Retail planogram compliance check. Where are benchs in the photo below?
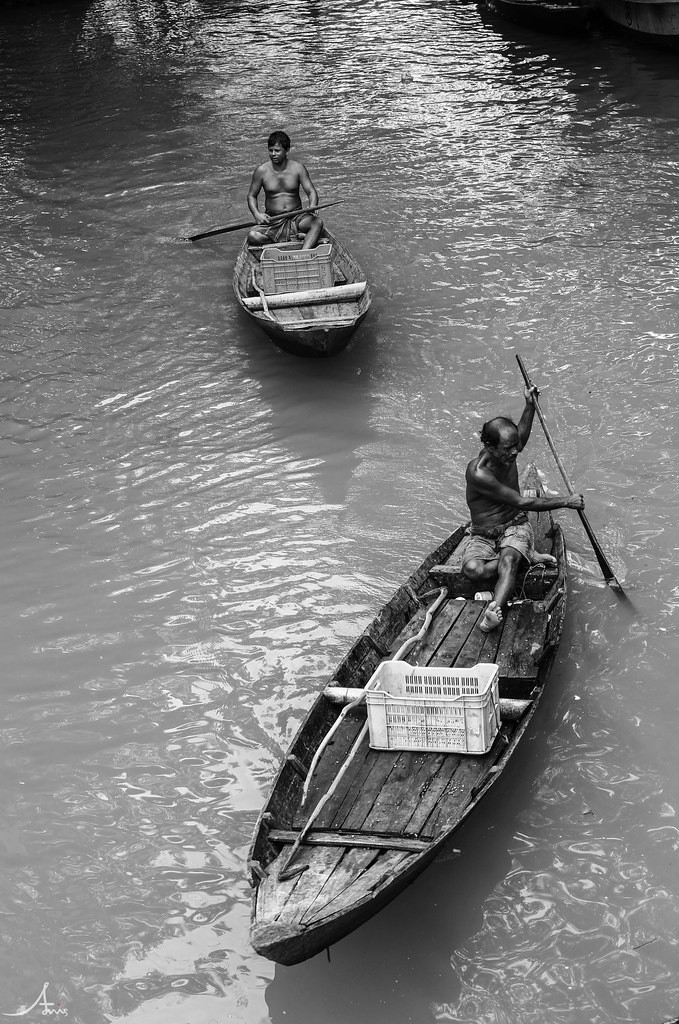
[243,281,366,314]
[248,237,327,264]
[430,558,558,579]
[323,683,532,718]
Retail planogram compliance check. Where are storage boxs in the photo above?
[261,243,332,296]
[365,661,500,754]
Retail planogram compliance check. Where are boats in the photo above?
[243,459,569,968]
[232,211,373,358]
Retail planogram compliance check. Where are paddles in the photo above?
[187,198,347,241]
[514,353,635,606]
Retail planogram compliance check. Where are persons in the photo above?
[246,131,325,260]
[459,379,586,633]
